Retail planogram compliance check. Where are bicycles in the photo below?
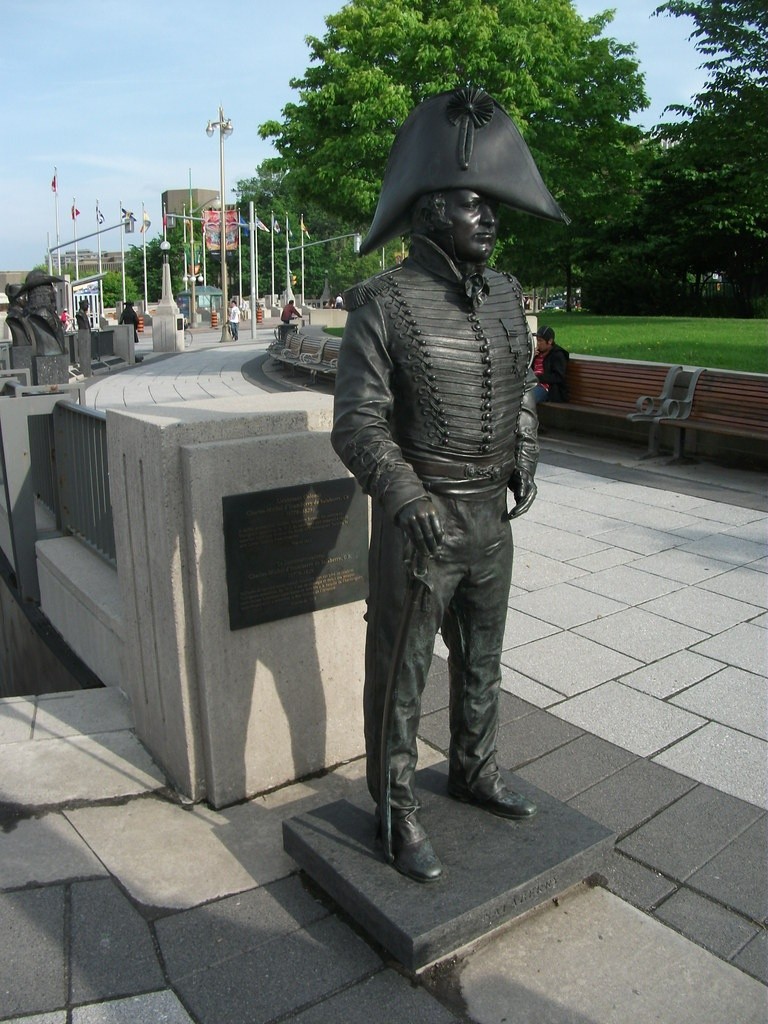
[184,329,193,348]
[273,316,303,342]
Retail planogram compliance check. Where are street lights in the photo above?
[205,102,234,343]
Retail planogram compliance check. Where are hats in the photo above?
[531,326,556,340]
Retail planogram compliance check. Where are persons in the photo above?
[227,300,240,340]
[527,326,570,405]
[61,308,70,332]
[119,301,143,363]
[332,86,573,886]
[76,299,91,332]
[329,292,344,308]
[281,300,303,324]
[4,270,69,356]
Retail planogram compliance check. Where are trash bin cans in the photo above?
[151,314,185,352]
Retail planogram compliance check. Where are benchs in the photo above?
[537,352,683,461]
[652,368,768,468]
[265,324,343,388]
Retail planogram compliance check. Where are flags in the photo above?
[122,207,137,221]
[186,219,191,231]
[51,171,56,193]
[95,204,105,224]
[239,216,249,237]
[288,223,293,237]
[257,217,270,232]
[141,209,151,232]
[72,205,80,219]
[300,219,310,239]
[273,218,280,233]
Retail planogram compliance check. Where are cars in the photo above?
[542,299,574,311]
